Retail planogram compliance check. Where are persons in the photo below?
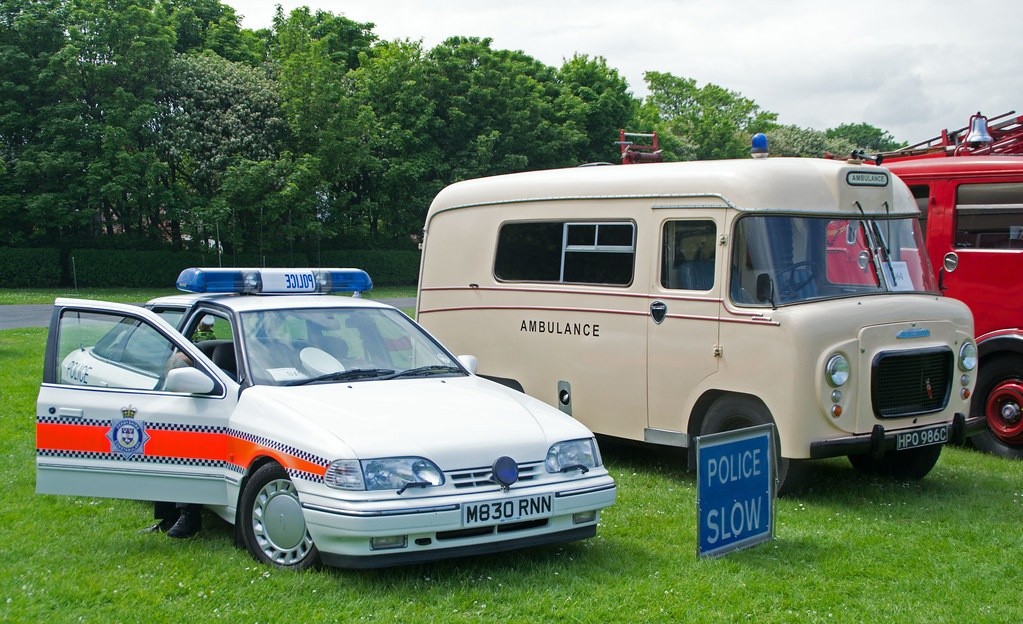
[142,352,238,537]
[191,315,218,342]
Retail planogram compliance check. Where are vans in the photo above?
[410,130,981,478]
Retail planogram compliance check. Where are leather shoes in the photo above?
[139,518,176,536]
[165,514,201,539]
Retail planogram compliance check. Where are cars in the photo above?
[33,269,621,570]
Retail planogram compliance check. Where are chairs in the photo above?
[678,260,740,302]
[784,269,818,301]
[211,342,236,374]
[320,336,349,361]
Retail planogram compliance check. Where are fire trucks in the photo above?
[820,109,1023,447]
